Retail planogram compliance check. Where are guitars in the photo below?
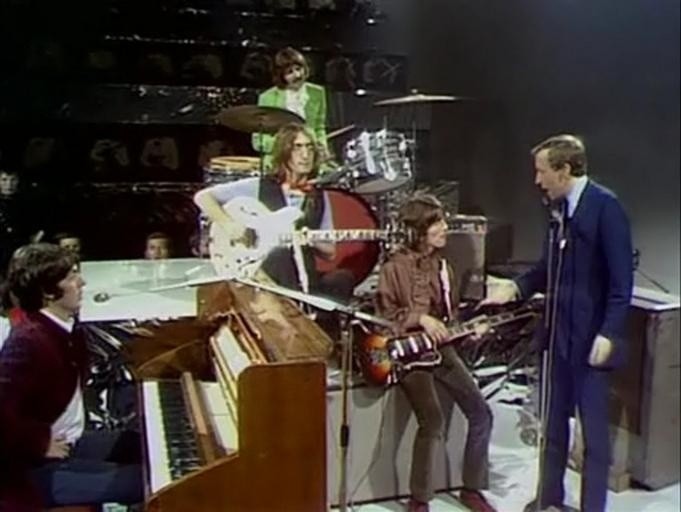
[359,298,547,385]
[211,196,393,280]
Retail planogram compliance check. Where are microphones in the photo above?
[91,285,134,305]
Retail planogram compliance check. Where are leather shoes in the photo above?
[408,499,429,512]
[461,489,497,512]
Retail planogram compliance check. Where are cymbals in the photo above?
[372,92,459,107]
[218,104,307,133]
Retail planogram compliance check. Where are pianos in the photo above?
[132,279,335,507]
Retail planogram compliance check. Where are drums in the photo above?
[343,130,413,192]
[205,156,264,183]
[313,189,380,288]
[444,212,488,301]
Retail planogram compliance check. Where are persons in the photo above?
[376,193,498,511]
[193,123,338,323]
[0,244,141,512]
[1,1,406,258]
[475,135,636,512]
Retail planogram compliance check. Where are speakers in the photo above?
[575,286,681,490]
